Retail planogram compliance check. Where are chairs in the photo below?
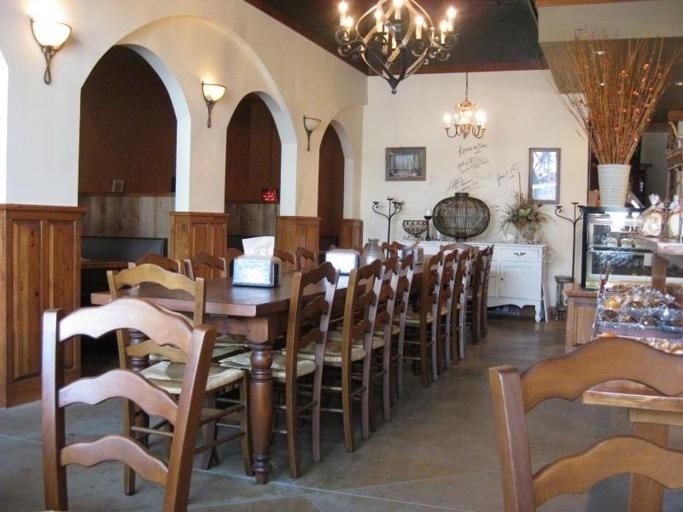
[489,337,683,512]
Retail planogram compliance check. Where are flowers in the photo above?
[500,193,554,227]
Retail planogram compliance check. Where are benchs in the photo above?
[81,235,168,289]
[319,236,339,263]
[227,235,261,276]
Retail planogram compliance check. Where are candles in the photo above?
[425,208,432,215]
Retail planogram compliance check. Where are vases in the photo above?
[526,227,544,245]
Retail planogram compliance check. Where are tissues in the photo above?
[232,233,281,289]
[325,248,359,274]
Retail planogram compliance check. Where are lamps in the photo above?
[334,0,488,139]
[30,19,72,84]
[201,83,225,128]
[261,118,280,203]
[302,116,322,151]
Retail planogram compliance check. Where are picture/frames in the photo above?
[385,146,426,180]
[112,179,124,193]
[528,147,560,204]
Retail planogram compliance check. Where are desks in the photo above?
[582,379,683,511]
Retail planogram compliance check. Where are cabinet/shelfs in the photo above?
[397,240,549,322]
[566,111,683,354]
